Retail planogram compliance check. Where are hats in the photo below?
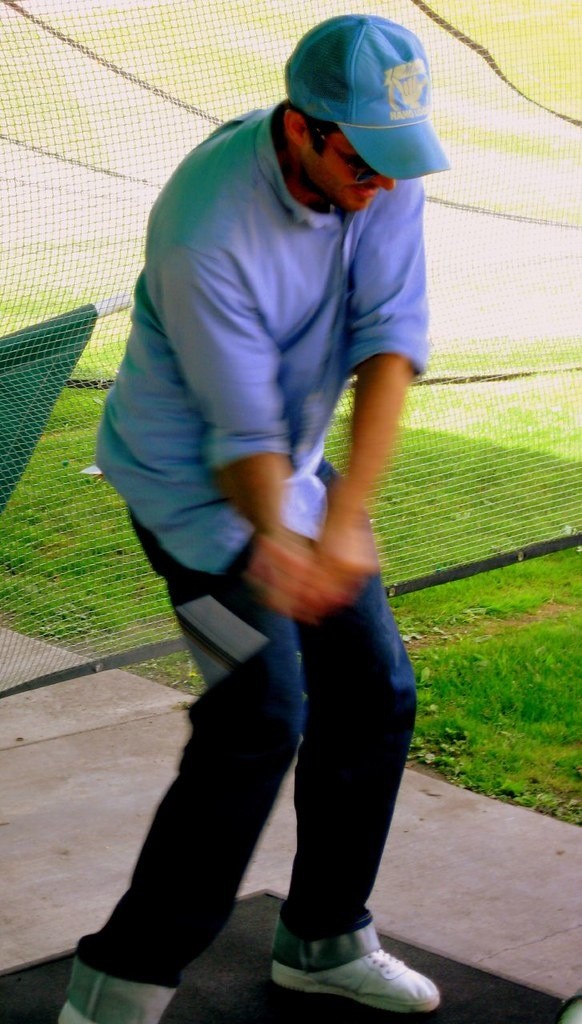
[284,14,452,180]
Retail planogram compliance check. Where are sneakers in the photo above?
[271,947,440,1015]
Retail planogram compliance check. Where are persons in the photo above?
[55,12,455,1024]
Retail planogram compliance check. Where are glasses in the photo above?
[312,124,381,184]
